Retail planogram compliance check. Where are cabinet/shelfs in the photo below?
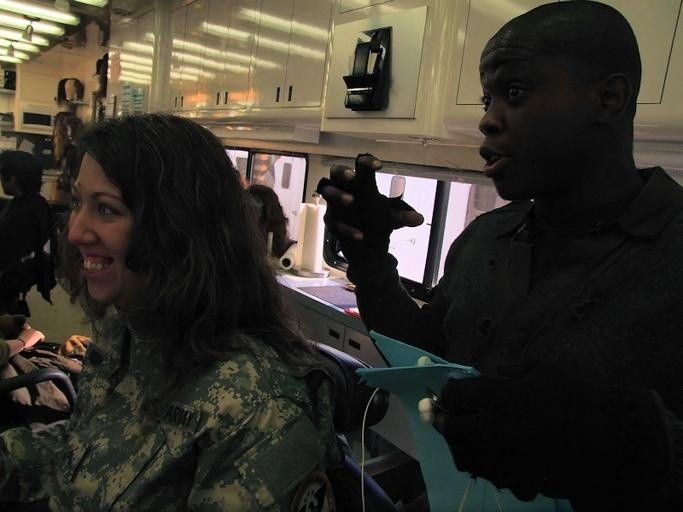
[15,20,102,117]
[162,0,209,126]
[434,0,683,145]
[278,286,389,371]
[209,0,255,120]
[255,0,336,114]
[106,3,162,140]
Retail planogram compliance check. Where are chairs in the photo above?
[297,337,402,508]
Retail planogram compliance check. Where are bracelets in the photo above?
[17,337,25,350]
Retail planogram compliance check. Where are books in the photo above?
[354,329,572,512]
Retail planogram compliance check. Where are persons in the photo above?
[53,110,87,182]
[0,320,71,415]
[0,112,336,512]
[316,0,682,505]
[246,182,290,249]
[0,148,53,265]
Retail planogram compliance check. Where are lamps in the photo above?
[20,14,42,42]
